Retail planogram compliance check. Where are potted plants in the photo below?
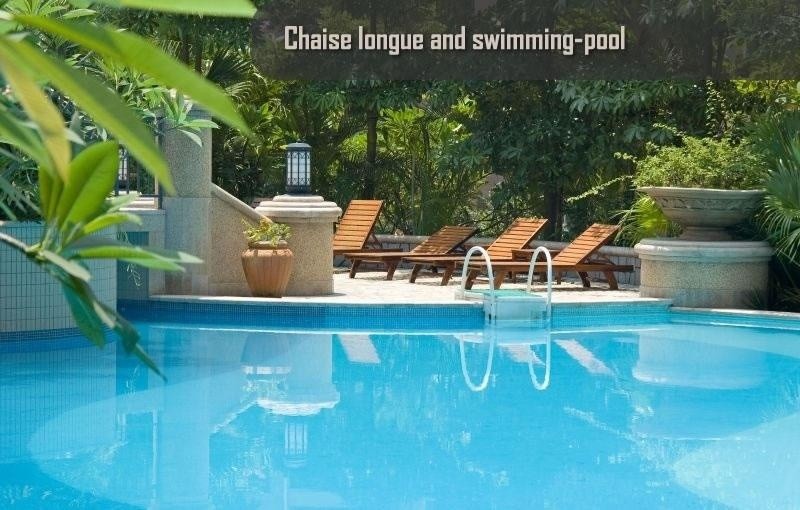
[240,216,293,297]
[564,81,767,243]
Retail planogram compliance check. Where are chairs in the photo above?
[337,334,381,365]
[503,343,543,365]
[333,198,634,291]
[556,338,607,371]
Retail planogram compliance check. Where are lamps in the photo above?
[283,417,309,469]
[116,143,129,186]
[285,142,312,194]
